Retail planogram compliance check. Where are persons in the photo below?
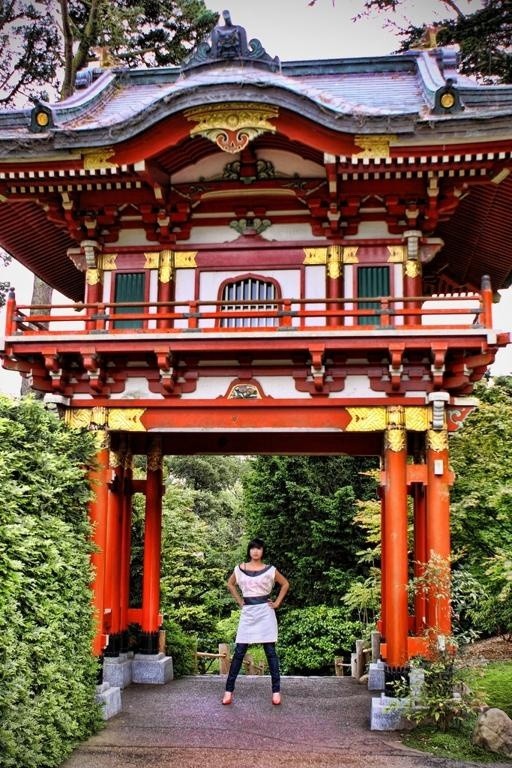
[219,537,291,706]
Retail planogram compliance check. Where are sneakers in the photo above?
[222,695,232,704]
[273,694,281,705]
